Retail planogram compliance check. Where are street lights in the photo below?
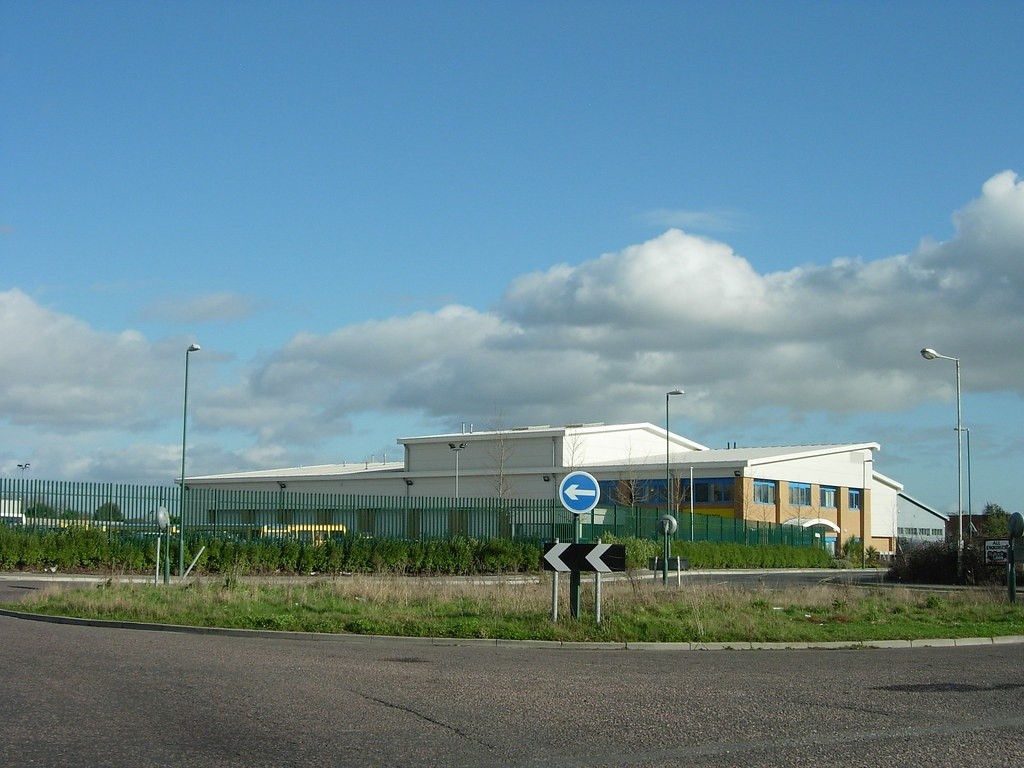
[919,346,965,553]
[448,441,467,498]
[666,389,685,557]
[954,427,975,547]
[176,342,202,580]
